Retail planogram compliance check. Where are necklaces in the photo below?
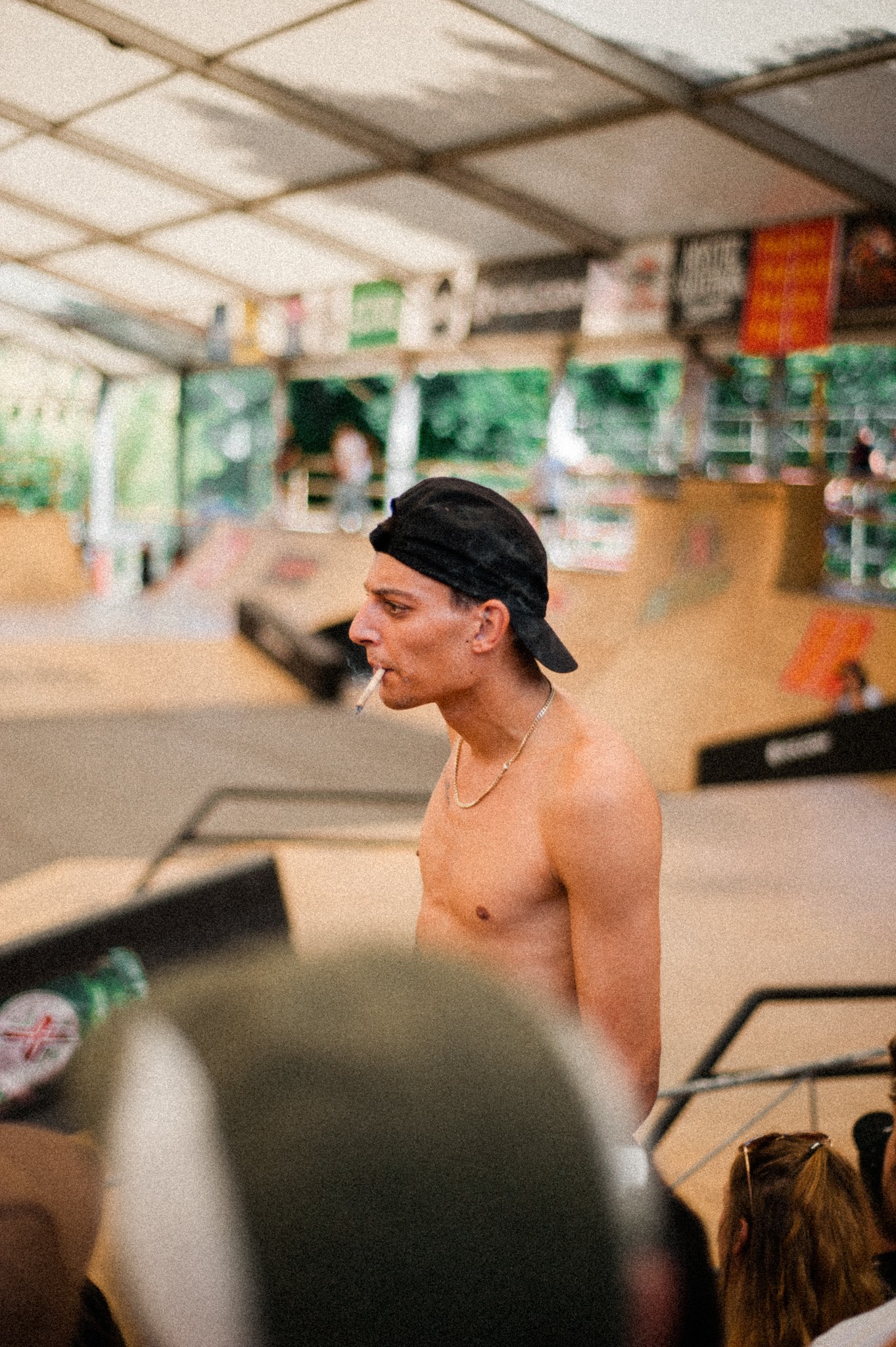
[453,675,555,809]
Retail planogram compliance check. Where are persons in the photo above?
[76,931,670,1347]
[349,480,664,1139]
[820,662,879,716]
[714,1133,885,1346]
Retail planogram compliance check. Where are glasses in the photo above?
[738,1130,830,1216]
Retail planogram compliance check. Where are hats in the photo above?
[62,937,636,1345]
[367,476,580,676]
